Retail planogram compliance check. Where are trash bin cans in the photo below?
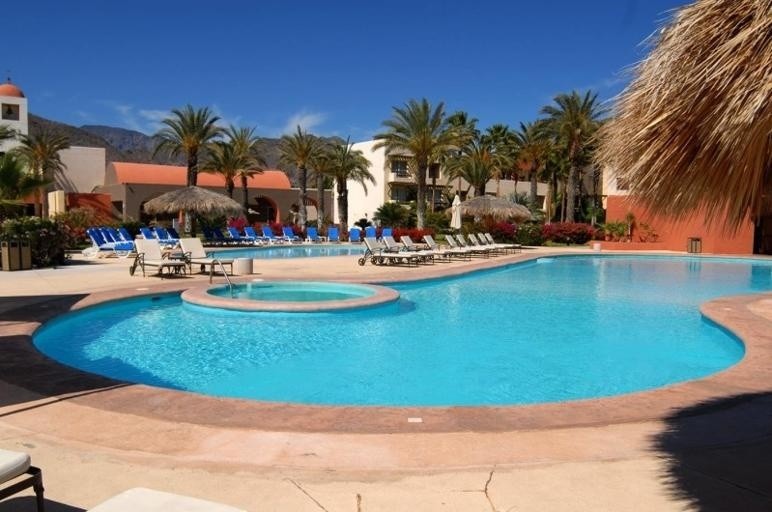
[687,236,702,253]
[1,239,33,271]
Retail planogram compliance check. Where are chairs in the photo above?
[228,224,392,249]
[86,224,236,279]
[356,229,522,267]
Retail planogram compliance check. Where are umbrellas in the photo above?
[450,195,461,230]
